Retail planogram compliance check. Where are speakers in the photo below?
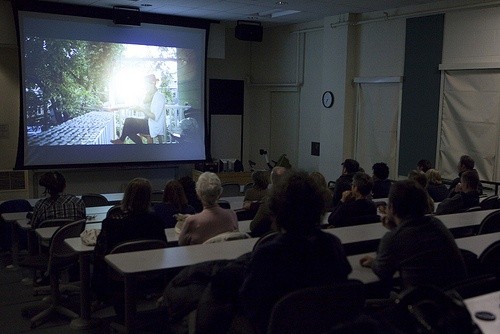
[114,9,141,26]
[235,24,264,41]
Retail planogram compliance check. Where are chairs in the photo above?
[268,284,355,334]
[20,218,86,331]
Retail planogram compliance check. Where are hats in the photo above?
[341,159,359,171]
[141,74,160,83]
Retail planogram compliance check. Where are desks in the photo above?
[0,169,500,334]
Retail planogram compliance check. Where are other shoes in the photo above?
[110,139,124,144]
[157,297,167,307]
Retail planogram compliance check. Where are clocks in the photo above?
[322,91,334,108]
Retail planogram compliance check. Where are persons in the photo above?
[151,178,196,228]
[18,171,88,281]
[359,180,472,301]
[436,170,480,215]
[233,167,353,334]
[110,74,166,144]
[177,176,203,214]
[242,154,483,235]
[90,177,168,319]
[178,171,239,247]
[328,172,377,228]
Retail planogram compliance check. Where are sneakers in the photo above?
[23,274,43,286]
